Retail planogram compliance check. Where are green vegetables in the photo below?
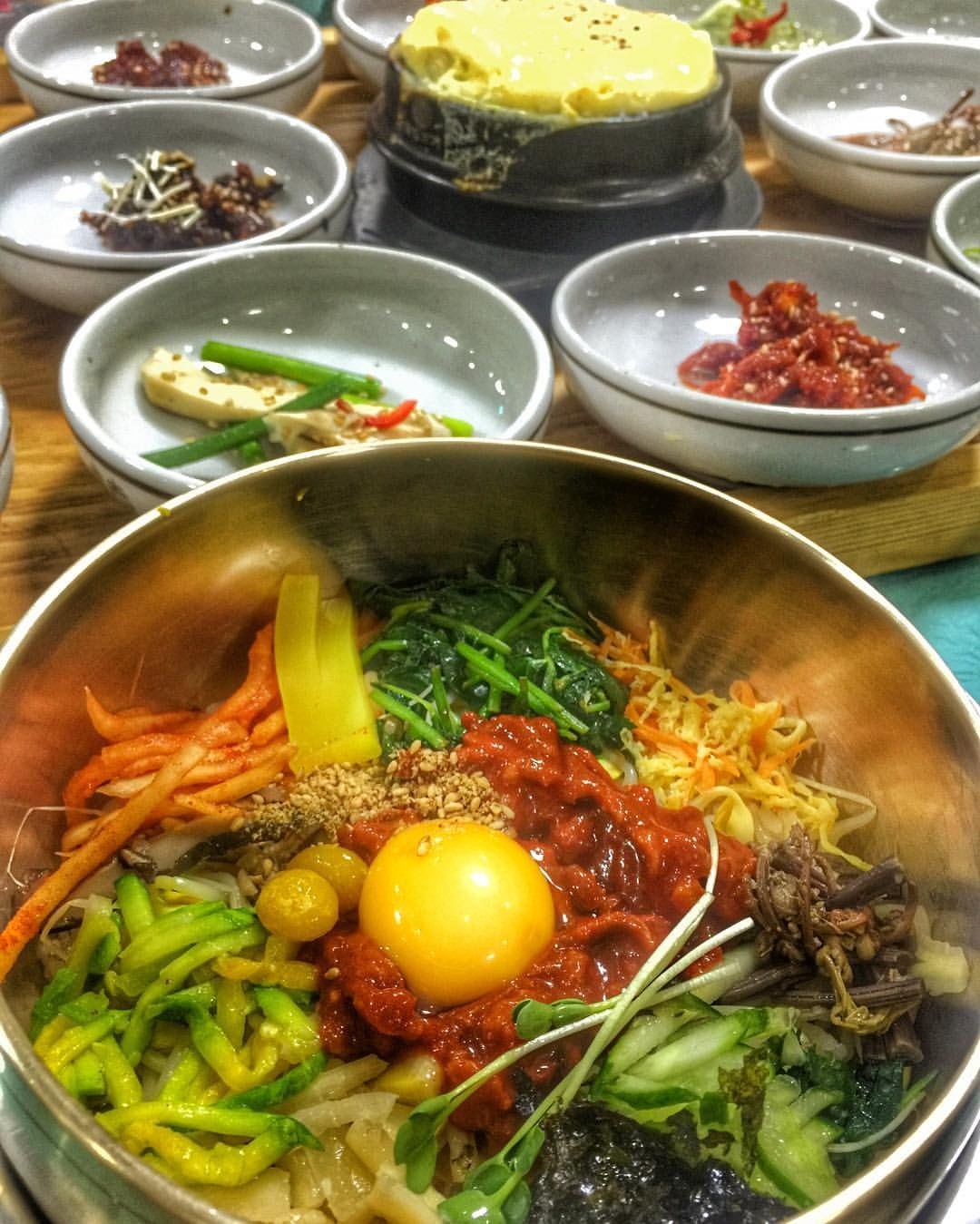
[134,337,383,469]
[341,535,638,754]
[393,811,939,1223]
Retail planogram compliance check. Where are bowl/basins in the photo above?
[1,439,980,1222]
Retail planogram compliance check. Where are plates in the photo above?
[624,0,871,111]
[871,0,980,282]
[0,100,359,314]
[760,36,980,219]
[337,0,422,95]
[554,227,980,485]
[7,2,324,114]
[59,239,554,517]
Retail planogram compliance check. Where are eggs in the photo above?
[359,816,557,1013]
